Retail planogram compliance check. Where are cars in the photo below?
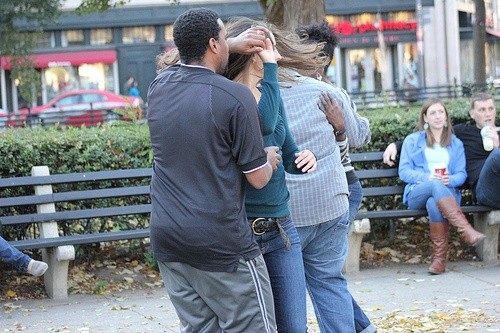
[7,91,143,127]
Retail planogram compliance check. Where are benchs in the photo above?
[0,152,500,297]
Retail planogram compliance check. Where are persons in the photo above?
[123,75,143,103]
[147,9,282,333]
[256,67,371,333]
[398,97,486,275]
[296,22,379,333]
[155,16,331,333]
[383,92,500,210]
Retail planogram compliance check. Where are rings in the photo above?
[257,30,260,35]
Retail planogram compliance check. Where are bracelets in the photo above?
[333,128,346,137]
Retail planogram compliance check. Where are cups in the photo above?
[480,126,494,151]
[434,168,446,176]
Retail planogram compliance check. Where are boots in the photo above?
[437,196,486,246]
[429,222,448,274]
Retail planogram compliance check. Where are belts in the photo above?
[346,170,355,178]
[249,217,291,250]
